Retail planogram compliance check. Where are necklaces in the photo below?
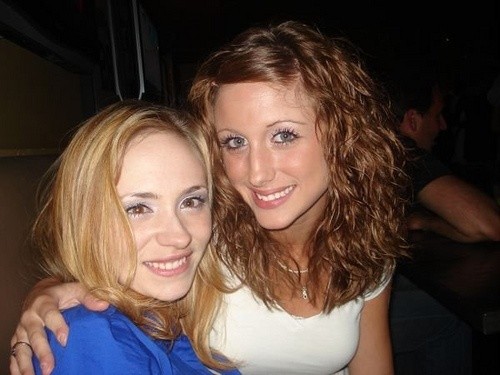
[273,255,326,302]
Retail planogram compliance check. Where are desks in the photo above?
[397,230,499,309]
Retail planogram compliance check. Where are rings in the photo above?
[10,341,35,361]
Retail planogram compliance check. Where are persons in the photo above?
[10,21,417,375]
[381,68,500,375]
[31,102,242,375]
